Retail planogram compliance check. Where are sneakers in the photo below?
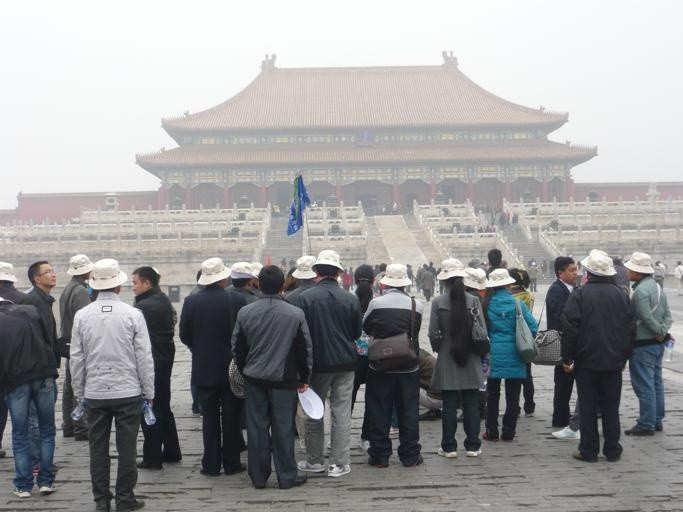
[11,486,32,498]
[327,462,353,478]
[39,481,58,494]
[297,458,325,473]
[572,448,597,463]
[465,447,482,457]
[625,423,654,437]
[437,447,458,458]
[94,500,111,511]
[651,422,663,433]
[404,454,424,466]
[367,457,389,468]
[551,426,581,440]
[419,409,441,422]
[32,465,59,477]
[482,432,499,442]
[359,437,371,452]
[115,498,147,512]
[198,410,222,418]
[0,447,6,458]
[525,409,535,417]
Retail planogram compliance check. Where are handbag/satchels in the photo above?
[53,333,70,359]
[512,307,534,365]
[367,333,419,374]
[470,302,490,358]
[530,329,563,365]
[228,358,245,401]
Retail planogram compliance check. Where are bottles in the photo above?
[479,352,488,392]
[71,402,85,421]
[141,399,157,426]
[663,337,673,362]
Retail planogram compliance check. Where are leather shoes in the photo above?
[136,460,161,470]
[198,465,221,476]
[225,461,247,475]
[161,454,183,461]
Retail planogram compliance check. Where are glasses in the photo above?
[37,267,54,279]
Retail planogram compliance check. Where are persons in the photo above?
[476,198,509,234]
[0,254,181,510]
[179,250,682,490]
[378,199,399,214]
[273,197,293,212]
[312,201,318,207]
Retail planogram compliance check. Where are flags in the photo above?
[286,176,310,237]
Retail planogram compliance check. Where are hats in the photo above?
[229,262,258,279]
[622,252,656,275]
[486,266,518,288]
[296,387,325,423]
[579,248,618,278]
[88,257,128,291]
[250,262,265,277]
[65,253,94,277]
[0,261,19,283]
[379,262,412,288]
[292,254,318,280]
[197,256,232,286]
[436,258,467,281]
[312,249,345,274]
[462,267,488,291]
